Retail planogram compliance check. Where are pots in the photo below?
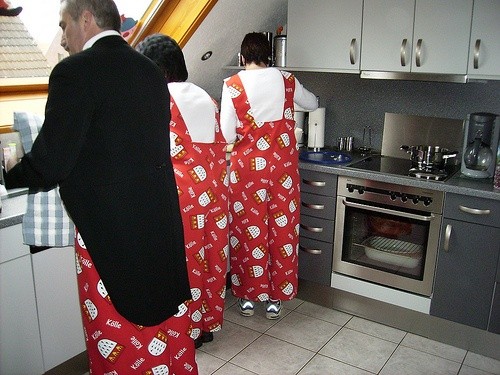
[399,143,460,178]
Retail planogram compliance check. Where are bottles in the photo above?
[338,136,354,152]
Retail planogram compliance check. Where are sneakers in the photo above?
[237,297,255,316]
[265,298,283,319]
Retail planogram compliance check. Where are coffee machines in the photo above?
[460,112,500,179]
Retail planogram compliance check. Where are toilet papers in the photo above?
[307,107,326,148]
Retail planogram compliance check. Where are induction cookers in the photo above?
[342,111,466,184]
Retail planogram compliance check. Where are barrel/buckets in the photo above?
[274,35,286,68]
[259,32,272,66]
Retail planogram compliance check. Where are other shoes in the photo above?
[200,331,213,342]
[194,338,202,349]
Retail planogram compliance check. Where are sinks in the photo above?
[342,155,456,188]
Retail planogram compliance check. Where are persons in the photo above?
[219,33,318,319]
[134,33,229,349]
[0,0,197,374]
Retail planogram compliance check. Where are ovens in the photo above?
[329,175,447,316]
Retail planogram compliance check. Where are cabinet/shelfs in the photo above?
[0,221,87,375]
[357,0,474,74]
[430,193,500,331]
[294,173,336,285]
[467,0,500,78]
[282,0,362,71]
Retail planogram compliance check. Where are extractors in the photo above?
[359,70,472,85]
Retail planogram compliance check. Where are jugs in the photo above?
[464,137,493,170]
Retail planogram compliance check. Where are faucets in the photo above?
[359,125,368,156]
